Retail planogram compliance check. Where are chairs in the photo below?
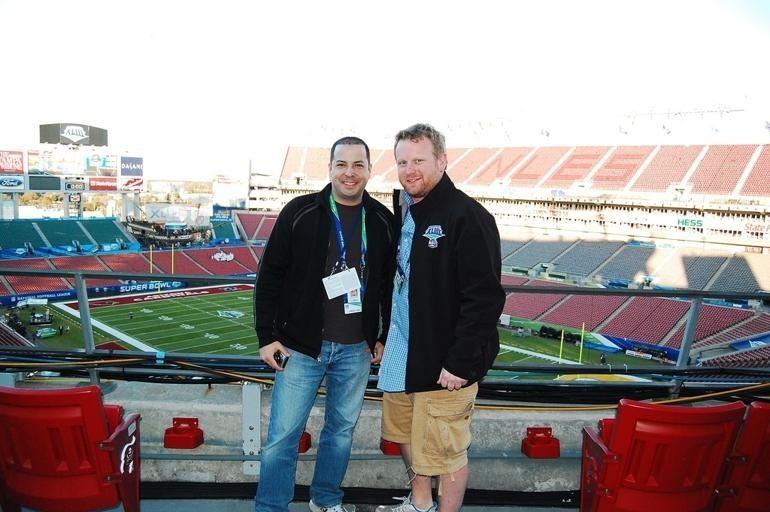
[579,398,770,512]
[0,386,142,512]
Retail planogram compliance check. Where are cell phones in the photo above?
[273,350,289,369]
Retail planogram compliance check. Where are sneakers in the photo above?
[375,493,437,512]
[309,499,343,512]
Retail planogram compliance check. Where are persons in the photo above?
[599,353,606,365]
[700,351,703,358]
[8,313,27,337]
[253,135,394,512]
[687,356,691,365]
[373,123,507,512]
[33,334,35,341]
[31,306,36,316]
[128,312,134,323]
[58,324,64,335]
[696,355,699,363]
[64,324,70,335]
